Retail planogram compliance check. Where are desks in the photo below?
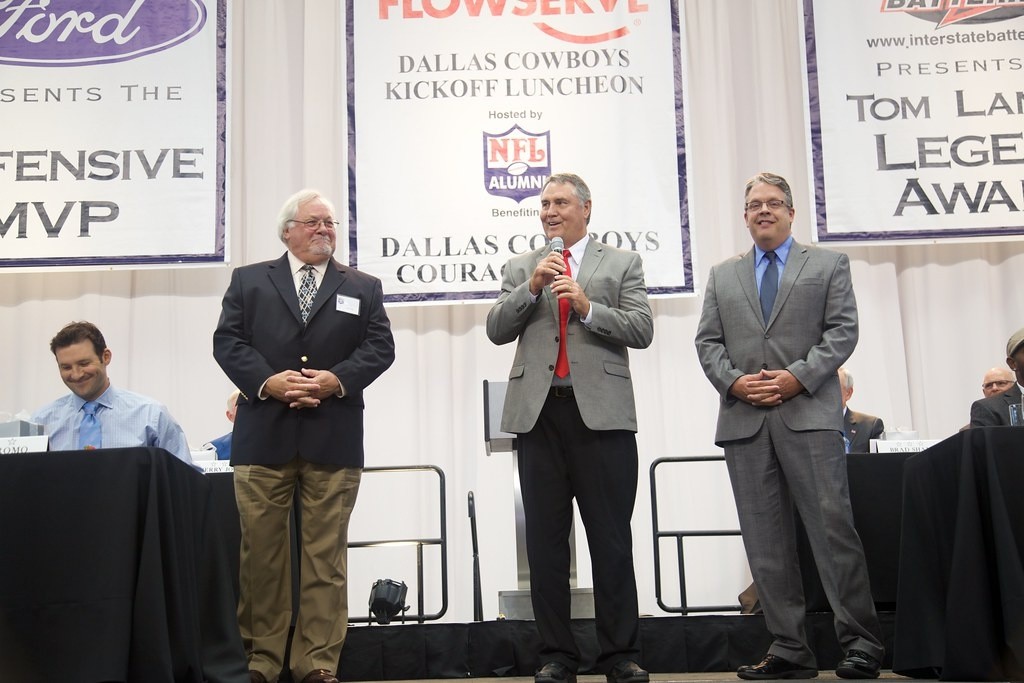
[795,425,1024,683]
[0,446,300,683]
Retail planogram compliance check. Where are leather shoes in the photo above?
[606,660,650,683]
[534,661,577,683]
[303,668,339,683]
[835,649,881,678]
[737,653,819,680]
[249,670,267,683]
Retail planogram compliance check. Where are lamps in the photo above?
[368,579,407,626]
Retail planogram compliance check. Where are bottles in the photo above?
[843,432,849,453]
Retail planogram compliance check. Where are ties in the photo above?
[77,401,102,450]
[554,250,572,378]
[760,252,779,327]
[298,265,318,328]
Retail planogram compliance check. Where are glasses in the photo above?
[746,200,791,211]
[982,380,1015,389]
[287,220,339,230]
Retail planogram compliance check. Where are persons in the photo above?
[212,189,396,683]
[969,327,1024,427]
[834,368,884,452]
[29,322,193,464]
[486,173,653,682]
[203,391,241,460]
[982,367,1015,397]
[695,173,886,680]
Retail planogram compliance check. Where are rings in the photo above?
[568,286,571,292]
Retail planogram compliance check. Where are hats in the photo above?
[1007,328,1024,357]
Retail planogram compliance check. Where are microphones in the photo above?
[551,236,564,281]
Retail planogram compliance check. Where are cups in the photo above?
[1008,403,1024,426]
